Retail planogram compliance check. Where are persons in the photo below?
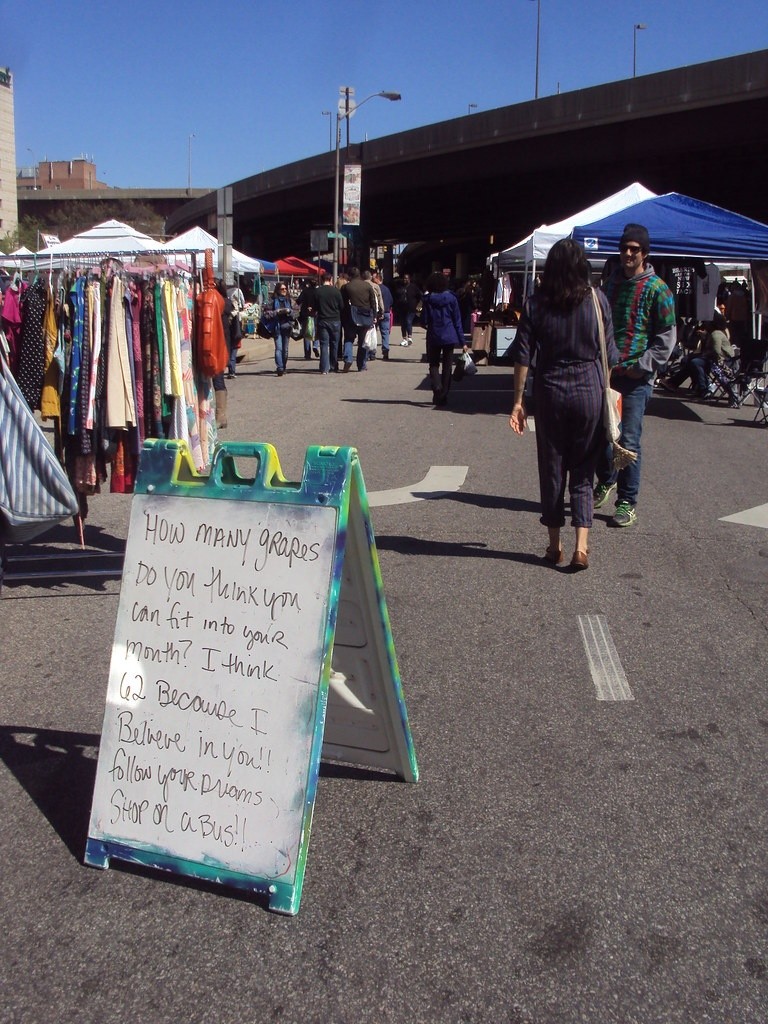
[509,239,617,570]
[592,223,677,527]
[659,311,731,399]
[213,279,245,429]
[251,267,393,376]
[395,268,468,405]
[344,204,359,223]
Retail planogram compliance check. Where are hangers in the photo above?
[0,249,215,284]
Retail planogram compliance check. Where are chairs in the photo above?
[654,345,768,426]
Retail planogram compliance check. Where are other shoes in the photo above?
[400,339,409,347]
[277,371,283,376]
[432,388,440,405]
[545,547,565,565]
[312,347,320,357]
[228,374,235,379]
[437,401,447,407]
[659,382,677,394]
[408,338,413,346]
[570,551,589,569]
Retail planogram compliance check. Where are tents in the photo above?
[0,219,325,318]
[568,192,768,339]
[494,182,755,340]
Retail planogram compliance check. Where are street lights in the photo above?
[189,133,196,189]
[632,23,648,79]
[26,148,37,191]
[468,103,478,115]
[331,89,402,286]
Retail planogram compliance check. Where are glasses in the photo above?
[617,244,643,254]
[280,288,288,290]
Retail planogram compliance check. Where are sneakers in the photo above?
[610,499,637,527]
[593,481,617,508]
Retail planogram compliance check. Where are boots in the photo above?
[215,389,228,431]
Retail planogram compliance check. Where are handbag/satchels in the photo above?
[602,387,638,469]
[361,323,377,355]
[453,358,466,382]
[305,317,315,342]
[291,318,305,342]
[459,352,478,377]
[257,316,278,341]
[195,248,230,380]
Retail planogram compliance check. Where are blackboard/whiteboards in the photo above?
[86,438,421,914]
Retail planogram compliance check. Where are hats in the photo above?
[620,224,650,253]
